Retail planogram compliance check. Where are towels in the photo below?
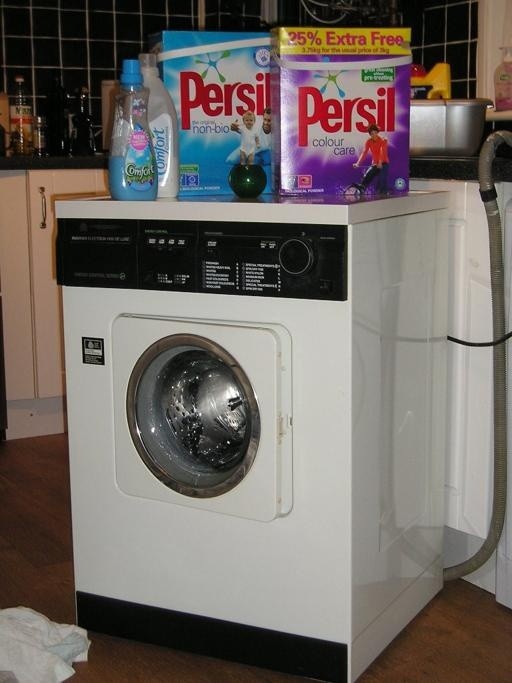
[0,605,92,683]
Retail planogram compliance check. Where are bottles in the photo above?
[65,86,96,156]
[232,197,266,204]
[10,76,47,157]
[228,164,268,198]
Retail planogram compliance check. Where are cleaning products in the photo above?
[271,26,413,197]
[162,32,277,197]
[109,59,158,200]
[136,50,181,199]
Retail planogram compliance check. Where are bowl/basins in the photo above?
[409,98,493,158]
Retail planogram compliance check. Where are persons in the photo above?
[352,124,395,191]
[234,109,260,166]
[226,107,272,165]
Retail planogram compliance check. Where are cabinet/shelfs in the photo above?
[0,169,114,440]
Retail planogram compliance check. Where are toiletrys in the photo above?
[493,45,512,110]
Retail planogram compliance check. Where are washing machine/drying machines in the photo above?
[50,191,452,653]
